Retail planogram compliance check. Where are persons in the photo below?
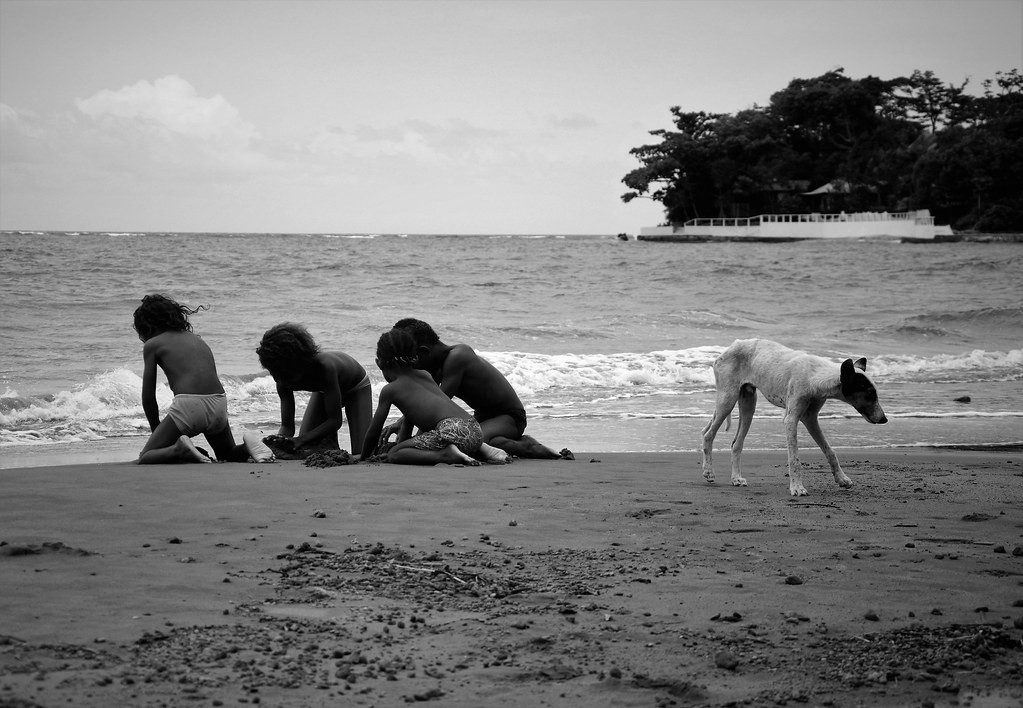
[256,322,372,455]
[353,318,561,466]
[133,294,276,463]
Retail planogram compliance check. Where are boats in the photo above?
[636,208,961,242]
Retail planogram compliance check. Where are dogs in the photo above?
[702,338,888,497]
